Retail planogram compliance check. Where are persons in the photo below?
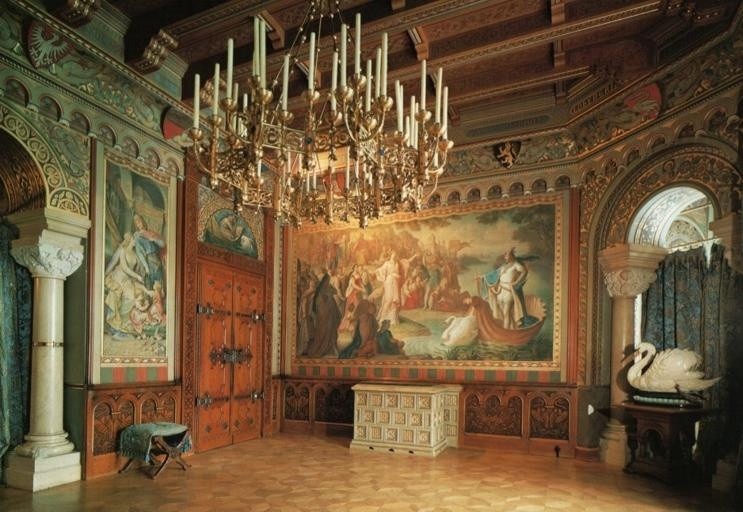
[295,228,529,360]
[108,230,138,332]
[128,212,166,303]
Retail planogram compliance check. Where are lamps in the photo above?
[190,0,453,230]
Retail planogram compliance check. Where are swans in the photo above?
[620,342,722,394]
[441,315,478,347]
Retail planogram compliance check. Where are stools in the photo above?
[118,428,192,480]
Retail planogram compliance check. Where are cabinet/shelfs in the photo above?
[614,401,716,485]
[349,384,464,457]
